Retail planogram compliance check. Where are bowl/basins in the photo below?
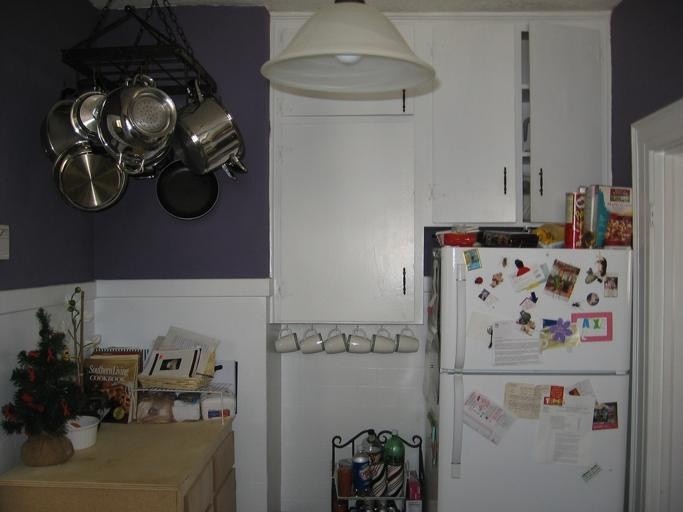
[66,415,99,449]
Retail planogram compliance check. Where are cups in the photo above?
[298,329,324,354]
[274,327,298,353]
[345,328,371,354]
[370,329,395,353]
[394,327,419,353]
[320,328,347,354]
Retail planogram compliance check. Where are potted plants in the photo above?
[63,285,100,451]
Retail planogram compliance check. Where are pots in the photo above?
[38,73,249,221]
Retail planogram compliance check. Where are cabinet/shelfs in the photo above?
[426,10,612,229]
[0,417,239,512]
[269,115,426,328]
[267,14,426,114]
[328,429,426,511]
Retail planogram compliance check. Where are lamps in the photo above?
[259,0,434,95]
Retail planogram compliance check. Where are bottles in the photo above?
[352,445,371,497]
[365,429,385,496]
[334,499,398,511]
[563,185,598,245]
[382,429,404,497]
[337,459,352,496]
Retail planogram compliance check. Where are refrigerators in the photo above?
[421,246,633,512]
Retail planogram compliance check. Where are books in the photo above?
[81,326,239,423]
